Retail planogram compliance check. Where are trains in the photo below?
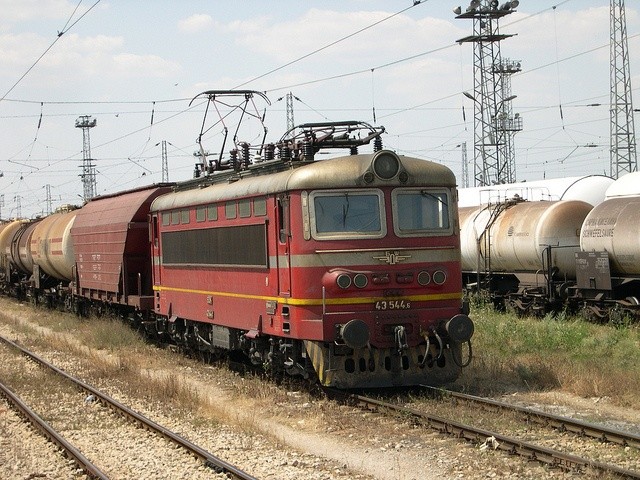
[0,120,476,397]
[438,195,640,328]
